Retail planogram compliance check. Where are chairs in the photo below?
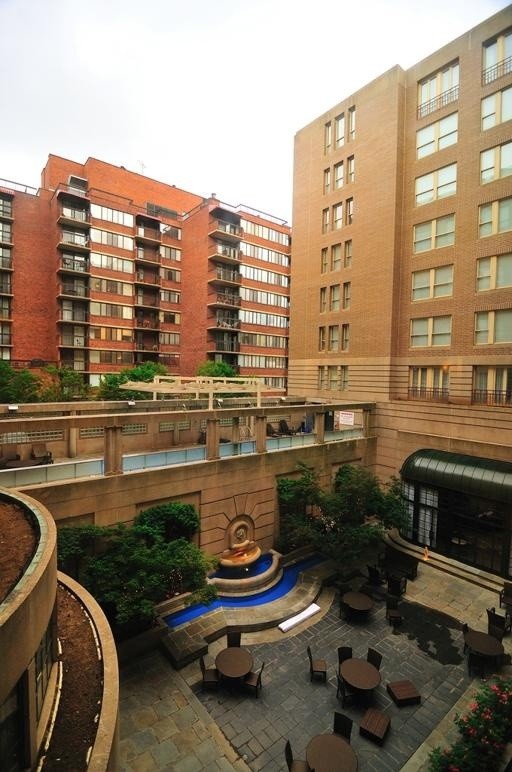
[484,580,511,642]
[282,739,308,771]
[196,654,217,693]
[466,650,485,679]
[331,711,352,744]
[383,571,406,626]
[306,642,382,709]
[266,419,299,438]
[0,444,53,469]
[244,661,265,698]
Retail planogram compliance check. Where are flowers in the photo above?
[434,672,511,772]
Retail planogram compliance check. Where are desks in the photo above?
[214,647,253,690]
[338,591,374,625]
[462,630,504,668]
[303,733,357,771]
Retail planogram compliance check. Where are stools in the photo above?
[359,708,390,748]
[385,681,423,707]
